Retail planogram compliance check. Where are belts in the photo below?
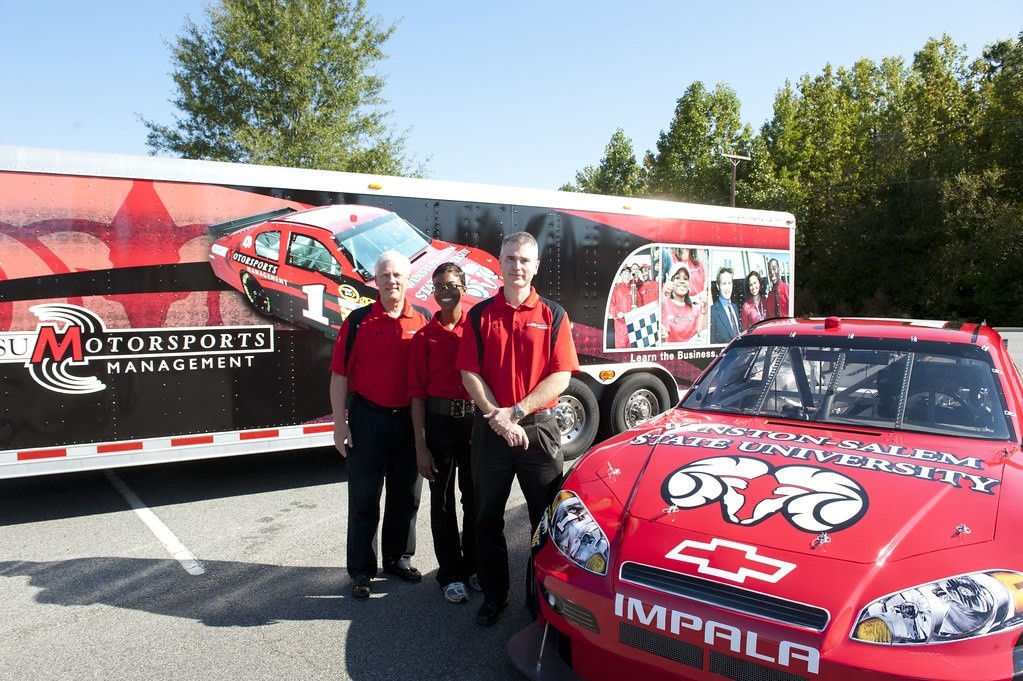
[348,391,415,417]
[425,397,477,419]
[518,408,554,425]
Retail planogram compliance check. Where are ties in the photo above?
[774,291,780,317]
[727,304,739,337]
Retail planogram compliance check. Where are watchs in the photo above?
[514,404,525,420]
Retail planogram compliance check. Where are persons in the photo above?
[767,258,789,319]
[670,248,706,315]
[330,249,422,602]
[455,231,580,628]
[407,262,482,603]
[711,267,740,344]
[661,262,708,343]
[609,263,659,348]
[741,271,767,330]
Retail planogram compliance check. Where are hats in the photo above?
[619,264,632,275]
[639,264,651,271]
[669,262,691,281]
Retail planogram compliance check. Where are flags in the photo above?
[624,300,659,348]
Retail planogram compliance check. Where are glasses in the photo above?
[432,283,464,293]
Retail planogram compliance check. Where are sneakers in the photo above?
[525,599,542,621]
[475,596,509,624]
[468,573,482,591]
[442,582,470,603]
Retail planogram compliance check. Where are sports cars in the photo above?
[208,206,504,340]
[528,316,1023,681]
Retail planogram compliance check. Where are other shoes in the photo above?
[351,576,372,601]
[383,565,422,583]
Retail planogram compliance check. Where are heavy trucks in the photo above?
[0,146,797,481]
